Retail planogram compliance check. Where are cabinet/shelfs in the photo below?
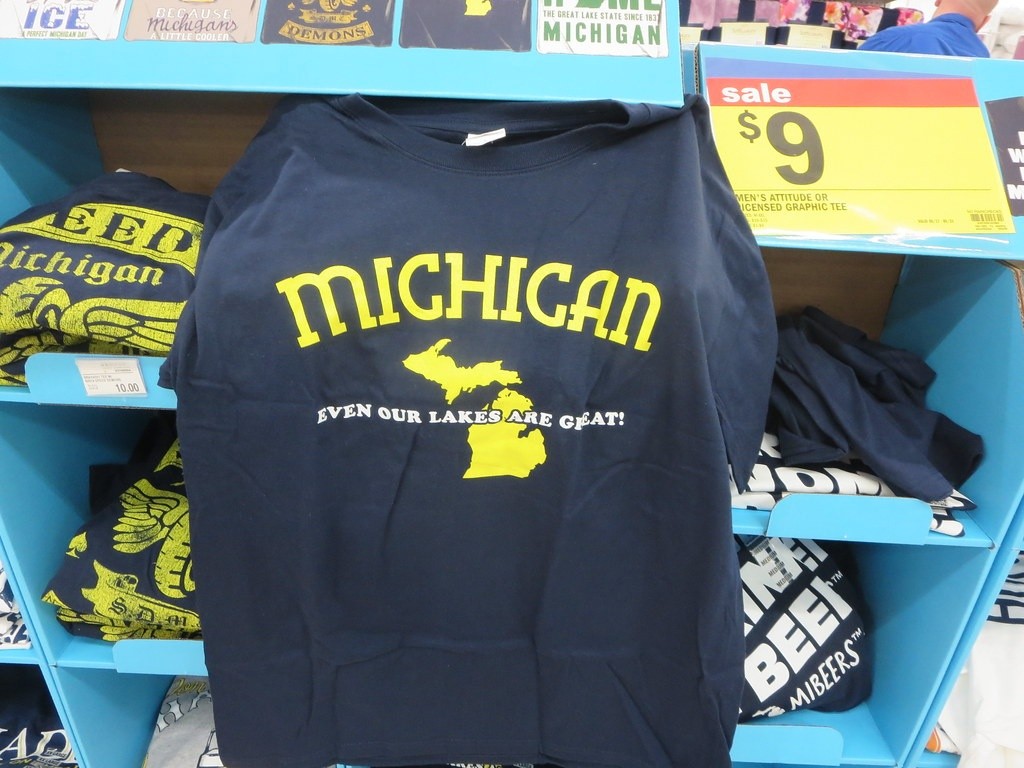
[0,8,1024,768]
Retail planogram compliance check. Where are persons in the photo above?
[858,0,999,58]
